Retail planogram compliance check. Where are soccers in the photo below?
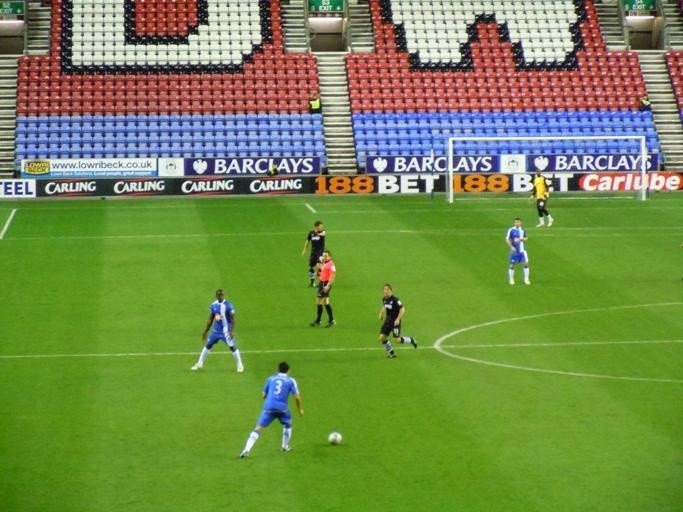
[329,433,341,445]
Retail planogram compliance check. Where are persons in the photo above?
[308,92,322,114]
[506,218,531,286]
[191,289,243,373]
[267,163,278,176]
[239,362,304,459]
[378,284,417,358]
[528,168,554,228]
[301,221,326,288]
[310,250,336,328]
[639,95,652,112]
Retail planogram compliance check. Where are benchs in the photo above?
[343,0,665,173]
[14,0,329,179]
[665,51,682,120]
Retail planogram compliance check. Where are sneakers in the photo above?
[524,278,531,285]
[410,336,417,348]
[281,445,292,454]
[324,320,336,328]
[509,278,515,286]
[237,365,244,373]
[190,363,202,371]
[548,218,554,227]
[240,449,249,458]
[310,320,321,327]
[536,223,545,228]
[385,354,396,358]
[309,282,319,288]
